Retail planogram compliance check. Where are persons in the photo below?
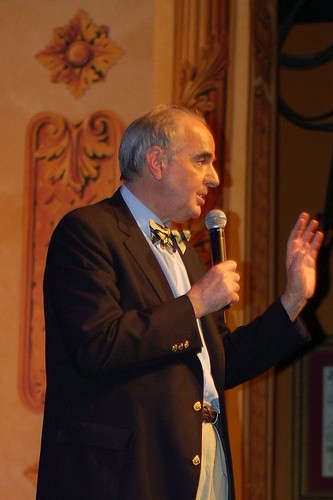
[35,104,322,500]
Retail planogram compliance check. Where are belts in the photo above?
[201,403,225,428]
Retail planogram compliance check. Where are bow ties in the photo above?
[148,219,192,257]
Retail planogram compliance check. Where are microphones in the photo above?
[205,209,227,266]
